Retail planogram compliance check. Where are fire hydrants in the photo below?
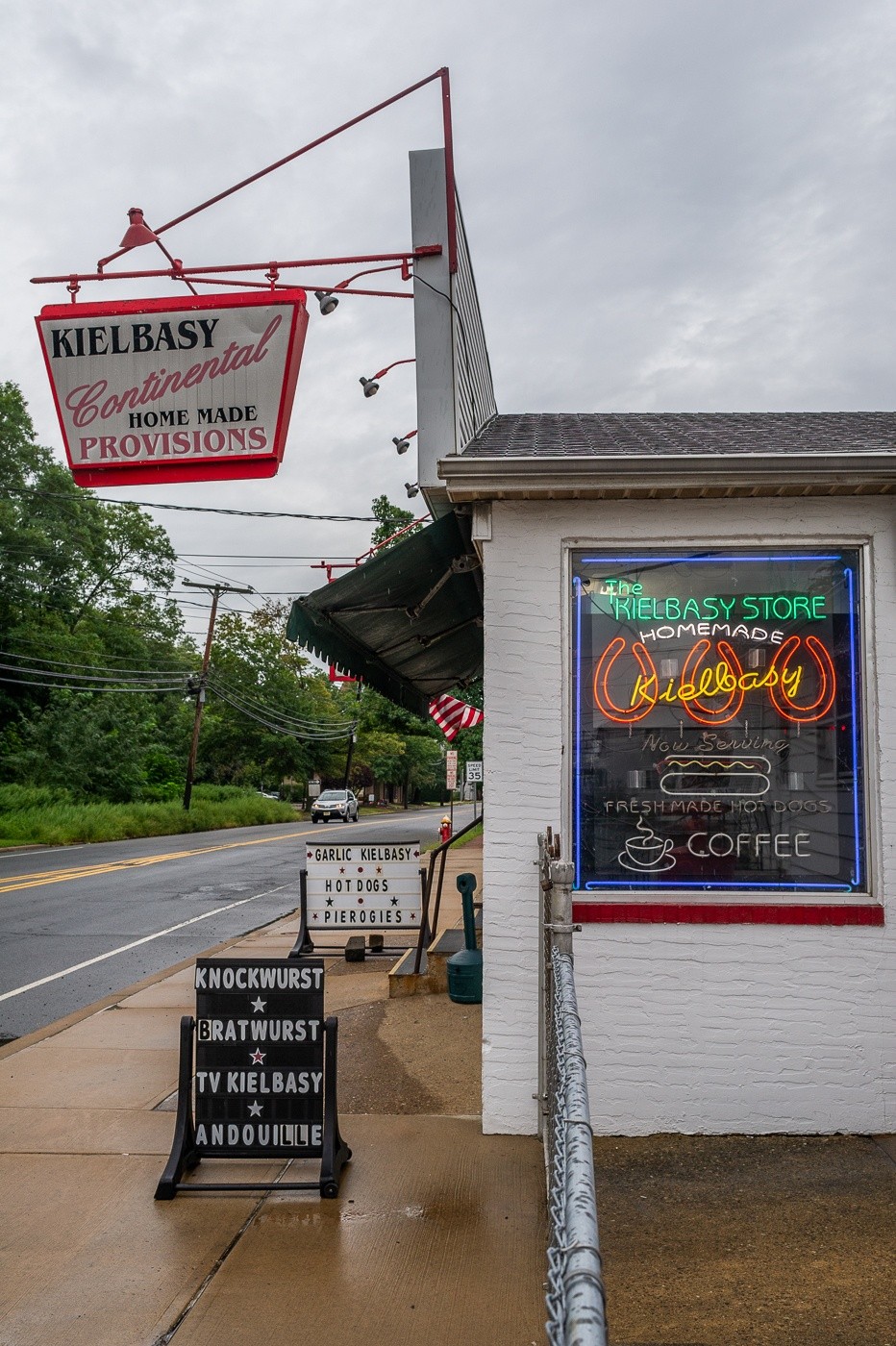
[438,815,452,844]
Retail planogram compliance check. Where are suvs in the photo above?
[310,789,359,824]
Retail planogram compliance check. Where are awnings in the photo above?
[285,508,484,722]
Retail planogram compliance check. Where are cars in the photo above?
[257,791,279,800]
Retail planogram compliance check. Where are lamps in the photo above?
[392,429,418,455]
[404,482,419,498]
[314,263,413,315]
[118,208,161,248]
[359,359,416,397]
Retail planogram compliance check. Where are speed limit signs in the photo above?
[465,761,483,782]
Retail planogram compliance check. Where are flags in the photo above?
[429,694,484,742]
